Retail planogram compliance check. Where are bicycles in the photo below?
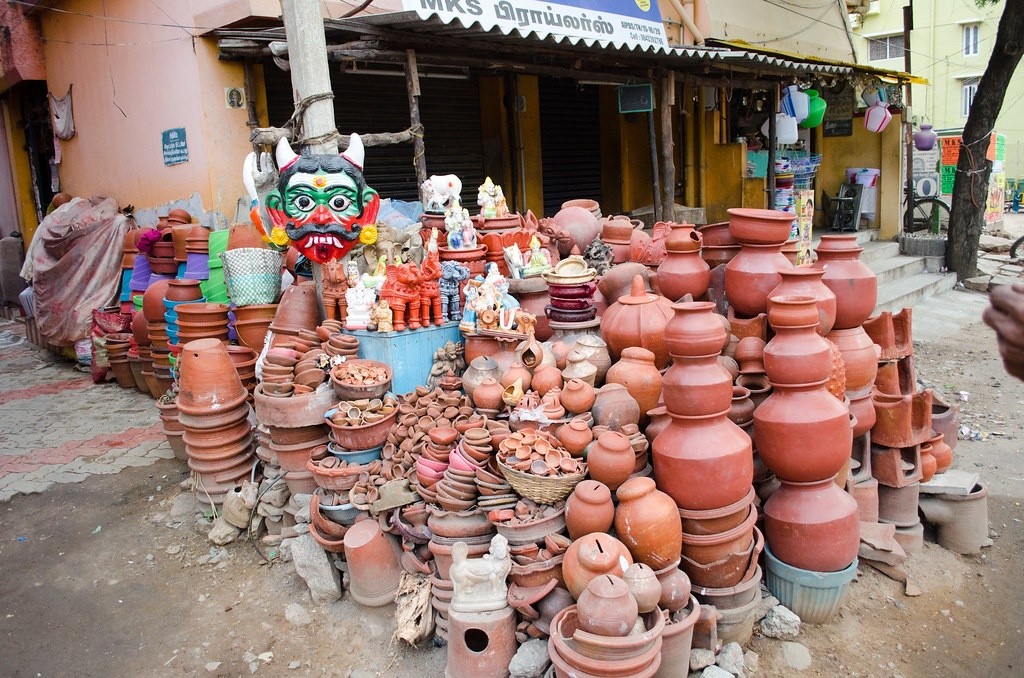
[903,188,952,235]
[1009,235,1024,259]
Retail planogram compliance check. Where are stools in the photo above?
[824,197,855,235]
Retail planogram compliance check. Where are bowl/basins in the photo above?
[262,318,359,398]
[330,358,393,399]
[697,216,741,246]
[428,534,498,644]
[677,484,765,648]
[847,167,880,188]
[702,245,741,269]
[327,442,382,465]
[324,401,399,448]
[354,386,586,520]
[306,457,371,491]
[726,208,798,244]
[756,541,859,622]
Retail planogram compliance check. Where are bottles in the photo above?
[664,301,727,357]
[918,432,952,483]
[863,101,893,133]
[551,207,633,263]
[652,403,753,511]
[800,89,827,128]
[762,322,830,384]
[658,224,710,300]
[861,84,888,107]
[824,325,878,399]
[848,391,876,438]
[763,473,860,572]
[766,268,836,336]
[810,235,877,328]
[779,85,810,124]
[662,350,733,415]
[914,125,937,151]
[753,377,851,483]
[560,379,692,637]
[724,243,794,316]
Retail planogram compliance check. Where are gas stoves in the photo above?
[832,183,864,232]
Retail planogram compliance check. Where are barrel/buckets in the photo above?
[343,521,406,607]
[103,209,284,504]
[761,113,798,144]
[254,381,336,503]
[271,285,318,335]
[546,604,665,678]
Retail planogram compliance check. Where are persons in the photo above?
[459,282,479,333]
[523,235,551,277]
[444,208,473,249]
[347,261,360,288]
[376,300,392,332]
[486,262,520,328]
[477,177,509,217]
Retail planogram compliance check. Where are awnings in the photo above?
[339,9,930,86]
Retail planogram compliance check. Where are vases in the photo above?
[104,204,323,514]
[420,484,765,678]
